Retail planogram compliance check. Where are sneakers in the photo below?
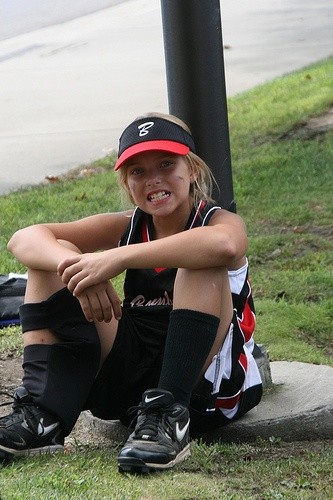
[0,397,66,457]
[117,389,190,474]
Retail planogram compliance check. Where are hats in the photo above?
[114,117,197,171]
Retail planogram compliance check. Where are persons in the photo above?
[1,110,265,474]
